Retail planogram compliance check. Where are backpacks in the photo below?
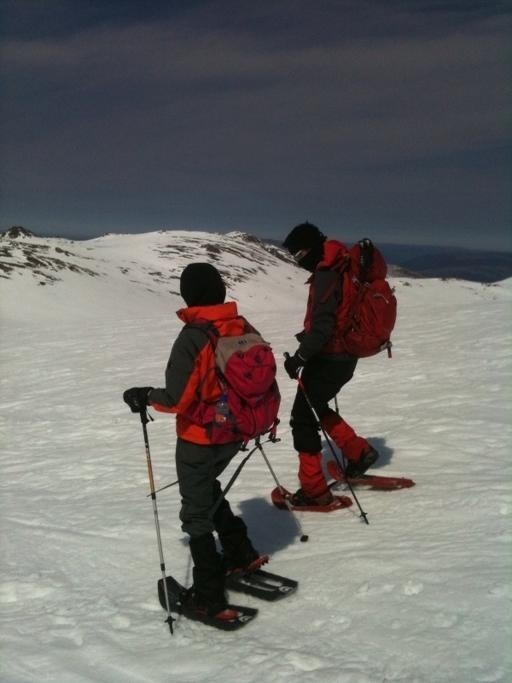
[330,237,398,364]
[187,316,280,445]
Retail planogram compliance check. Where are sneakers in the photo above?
[343,445,380,478]
[220,549,259,576]
[288,488,334,506]
[179,585,229,610]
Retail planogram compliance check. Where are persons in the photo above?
[119,260,280,622]
[280,219,384,512]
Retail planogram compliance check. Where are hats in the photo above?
[281,220,327,272]
[180,262,226,306]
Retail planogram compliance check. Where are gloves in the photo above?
[284,354,303,380]
[123,385,155,416]
